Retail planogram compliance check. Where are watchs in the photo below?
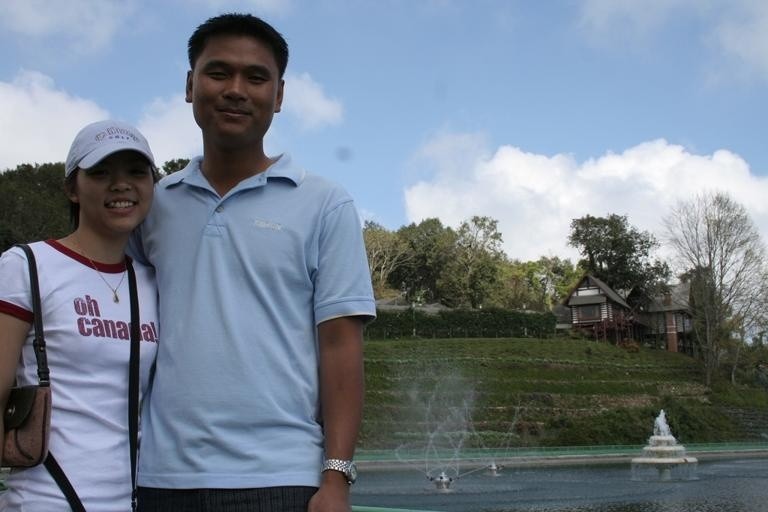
[321,458,360,486]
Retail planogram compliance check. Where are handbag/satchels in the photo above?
[1,385,50,467]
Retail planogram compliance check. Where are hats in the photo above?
[65,120,157,178]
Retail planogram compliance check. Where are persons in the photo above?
[123,8,377,512]
[0,117,161,511]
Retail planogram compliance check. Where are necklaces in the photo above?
[70,233,130,304]
